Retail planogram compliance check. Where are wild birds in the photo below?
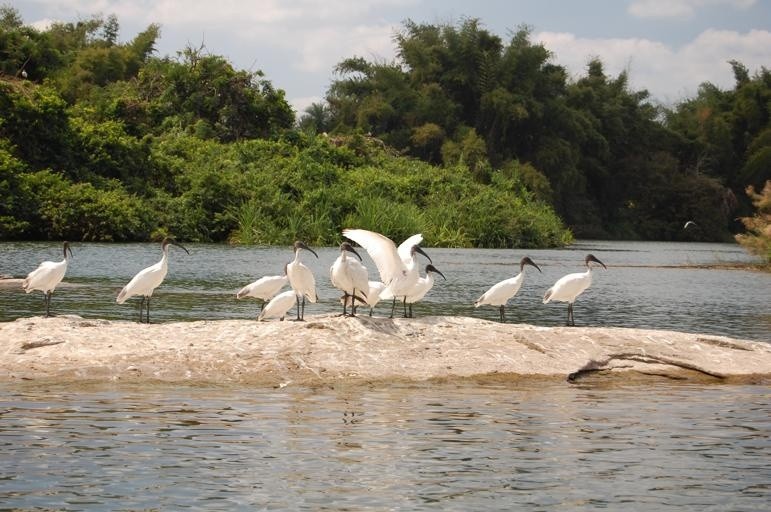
[20,241,73,319]
[472,256,542,323]
[236,274,290,312]
[542,254,607,326]
[256,290,298,322]
[115,237,190,324]
[329,229,447,319]
[284,241,318,322]
[684,221,696,228]
